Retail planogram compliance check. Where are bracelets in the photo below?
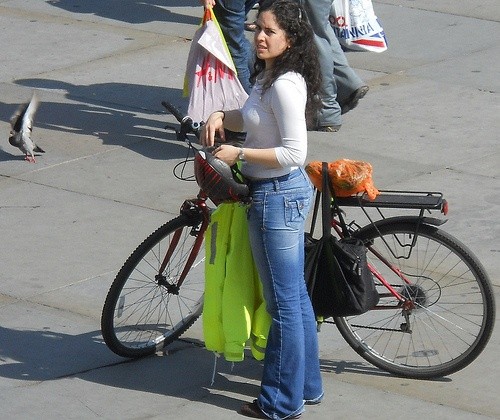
[217,111,226,121]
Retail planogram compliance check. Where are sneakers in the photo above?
[241,399,301,419]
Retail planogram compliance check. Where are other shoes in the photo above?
[245,22,258,31]
[307,124,341,132]
[340,86,369,115]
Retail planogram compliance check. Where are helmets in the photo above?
[194,150,250,204]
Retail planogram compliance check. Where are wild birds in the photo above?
[8,91,47,163]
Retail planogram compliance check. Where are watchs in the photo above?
[238,148,248,162]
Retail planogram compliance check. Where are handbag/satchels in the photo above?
[183,5,250,132]
[304,162,379,317]
[327,0,388,53]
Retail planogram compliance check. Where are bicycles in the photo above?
[100,99,497,380]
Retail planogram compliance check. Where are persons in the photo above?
[200,0,325,420]
[204,0,369,132]
